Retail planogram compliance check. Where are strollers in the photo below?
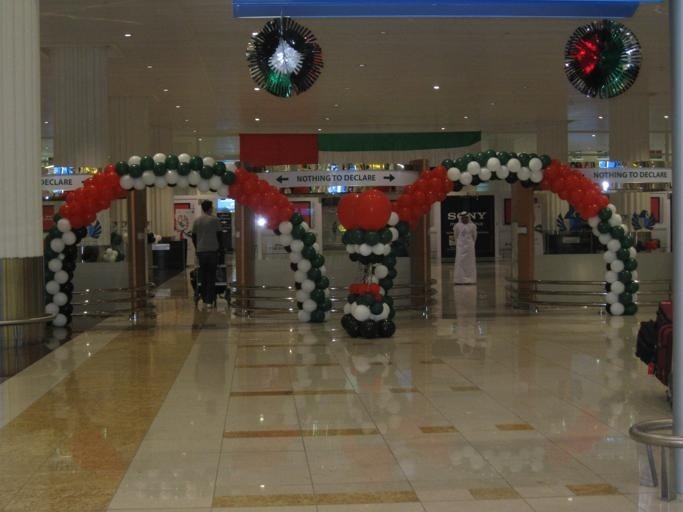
[191,249,231,304]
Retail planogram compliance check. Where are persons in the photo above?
[454,211,478,285]
[191,199,225,311]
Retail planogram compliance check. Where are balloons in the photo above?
[273,212,329,318]
[354,189,391,231]
[342,211,410,337]
[228,167,295,230]
[115,152,234,200]
[43,214,87,326]
[394,151,609,230]
[338,193,360,230]
[59,164,130,230]
[588,203,638,312]
